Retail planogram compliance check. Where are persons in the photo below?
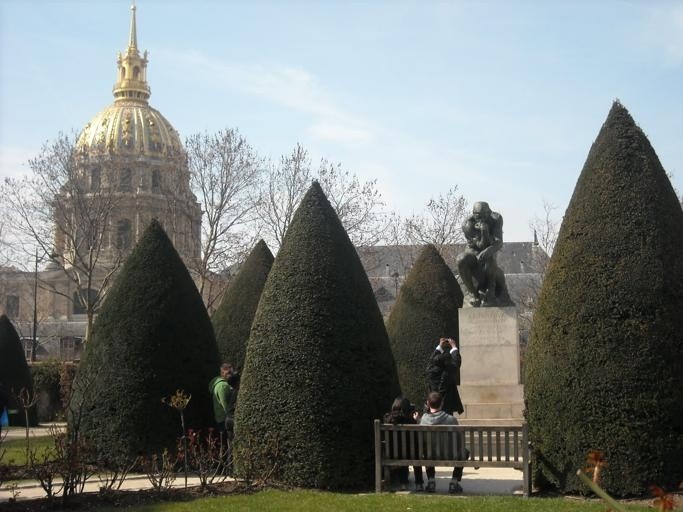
[424,337,464,417]
[418,392,469,493]
[209,363,233,475]
[455,201,503,306]
[223,374,240,476]
[380,396,423,492]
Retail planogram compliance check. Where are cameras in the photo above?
[444,338,450,344]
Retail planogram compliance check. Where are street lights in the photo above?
[31,246,63,362]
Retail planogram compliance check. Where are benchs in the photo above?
[374,418,529,499]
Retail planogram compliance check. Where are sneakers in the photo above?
[448,481,463,494]
[424,480,436,491]
[397,479,424,491]
